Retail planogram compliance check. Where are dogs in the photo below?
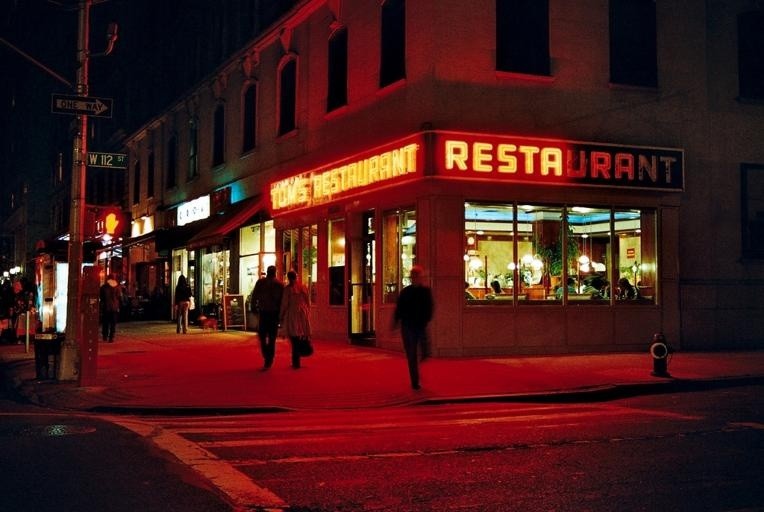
[198,316,219,336]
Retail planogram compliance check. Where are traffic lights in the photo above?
[100,207,122,237]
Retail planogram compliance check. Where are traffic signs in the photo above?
[50,93,112,119]
[86,152,128,170]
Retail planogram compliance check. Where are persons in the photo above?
[278,269,314,371]
[171,274,194,334]
[490,280,505,295]
[552,275,643,302]
[465,281,477,301]
[387,265,435,392]
[249,264,286,373]
[97,270,123,343]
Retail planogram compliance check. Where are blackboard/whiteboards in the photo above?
[224,295,246,327]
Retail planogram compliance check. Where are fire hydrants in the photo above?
[650,330,671,378]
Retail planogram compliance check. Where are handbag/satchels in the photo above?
[189,296,195,310]
[300,336,312,356]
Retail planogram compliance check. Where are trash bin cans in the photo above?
[34,332,65,380]
